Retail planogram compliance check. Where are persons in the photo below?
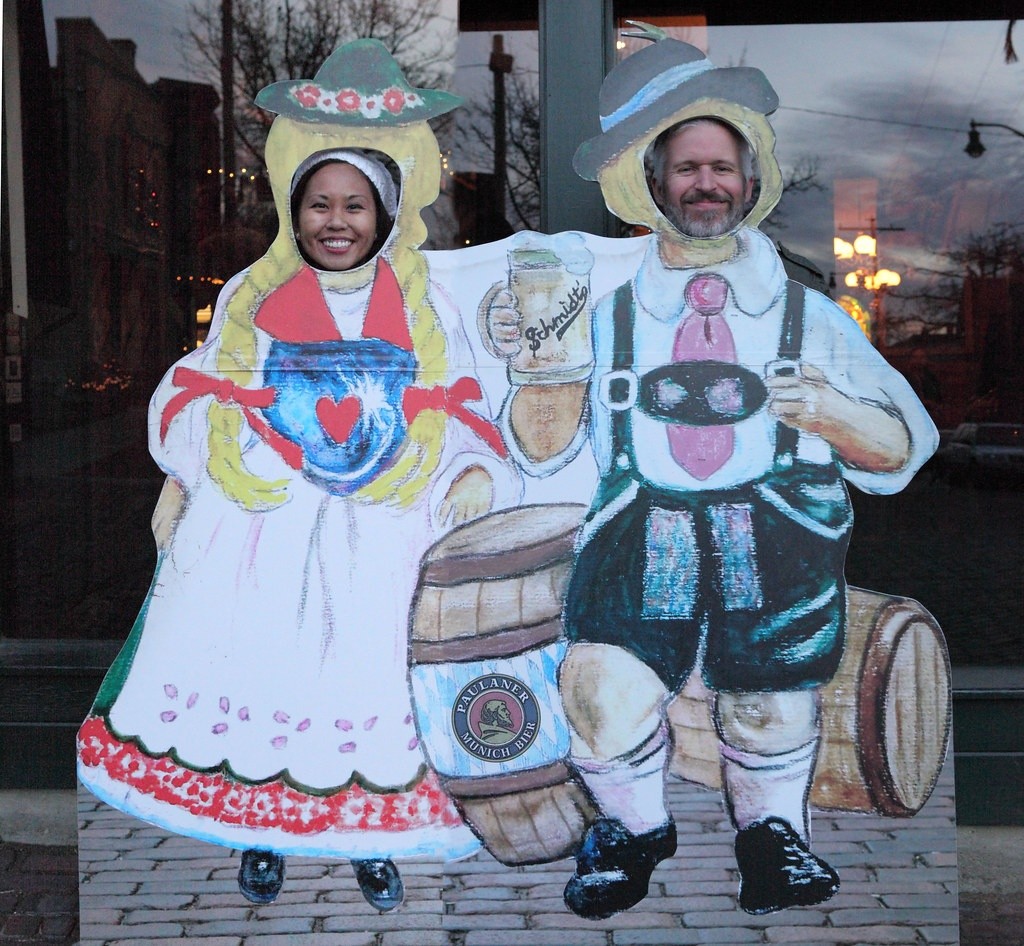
[652,118,756,237]
[903,348,939,398]
[290,149,398,271]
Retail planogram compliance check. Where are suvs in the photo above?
[945,422,1024,492]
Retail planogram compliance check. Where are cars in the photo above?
[923,428,955,479]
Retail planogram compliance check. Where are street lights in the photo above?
[484,31,512,248]
